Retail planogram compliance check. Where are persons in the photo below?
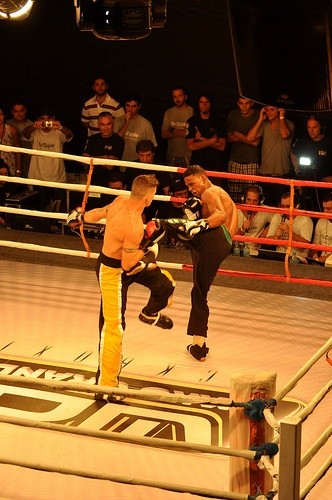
[23,108,73,232]
[265,191,313,264]
[186,93,226,188]
[97,170,146,224]
[113,92,158,172]
[80,75,125,169]
[79,112,126,210]
[290,114,332,212]
[226,89,264,204]
[141,165,238,362]
[233,186,275,260]
[6,101,34,175]
[67,174,176,402]
[0,100,22,193]
[0,164,25,231]
[311,196,332,266]
[161,84,194,166]
[148,181,189,247]
[247,98,295,207]
[125,139,172,221]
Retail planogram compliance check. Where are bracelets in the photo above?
[198,137,202,142]
[280,116,286,120]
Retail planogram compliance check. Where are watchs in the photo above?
[16,169,22,174]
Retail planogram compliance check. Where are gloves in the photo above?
[145,220,166,244]
[185,217,210,237]
[66,207,86,229]
[183,197,202,221]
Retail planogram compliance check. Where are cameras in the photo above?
[44,121,53,127]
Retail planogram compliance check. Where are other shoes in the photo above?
[139,309,174,329]
[187,342,210,362]
[94,394,126,402]
